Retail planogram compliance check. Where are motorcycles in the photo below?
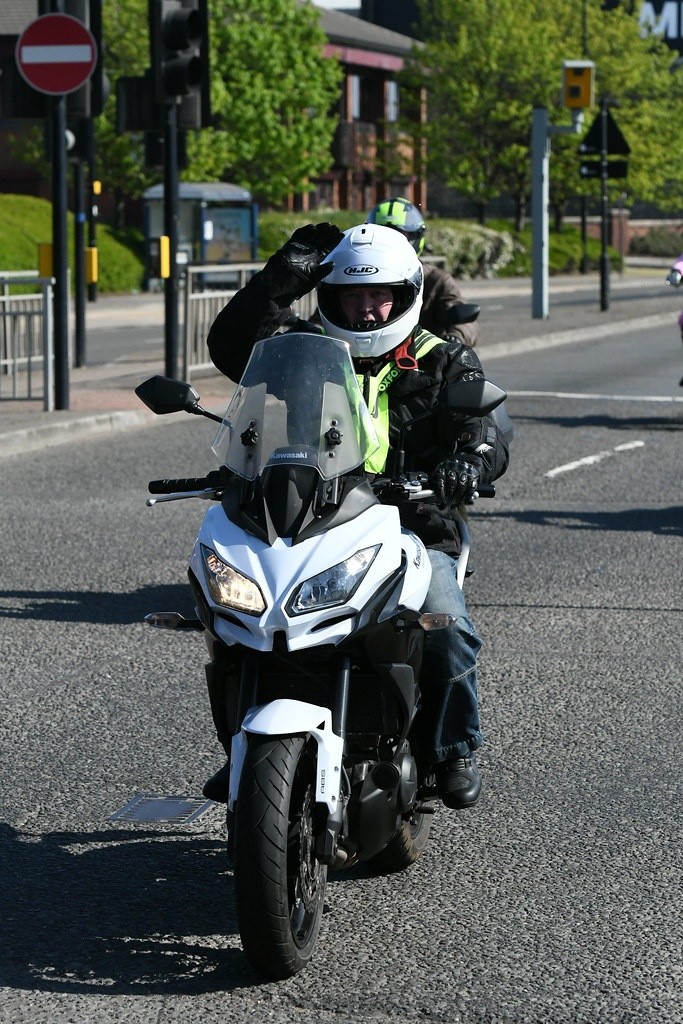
[132,333,515,980]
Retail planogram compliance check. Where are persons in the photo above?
[200,219,511,814]
[284,197,468,533]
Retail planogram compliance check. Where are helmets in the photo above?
[317,223,425,368]
[364,197,426,258]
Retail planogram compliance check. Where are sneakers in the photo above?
[202,760,230,804]
[436,750,482,809]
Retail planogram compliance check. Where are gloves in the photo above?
[251,221,345,307]
[429,451,484,510]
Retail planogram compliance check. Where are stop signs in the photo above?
[16,13,97,95]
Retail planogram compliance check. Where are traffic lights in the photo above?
[150,0,208,110]
